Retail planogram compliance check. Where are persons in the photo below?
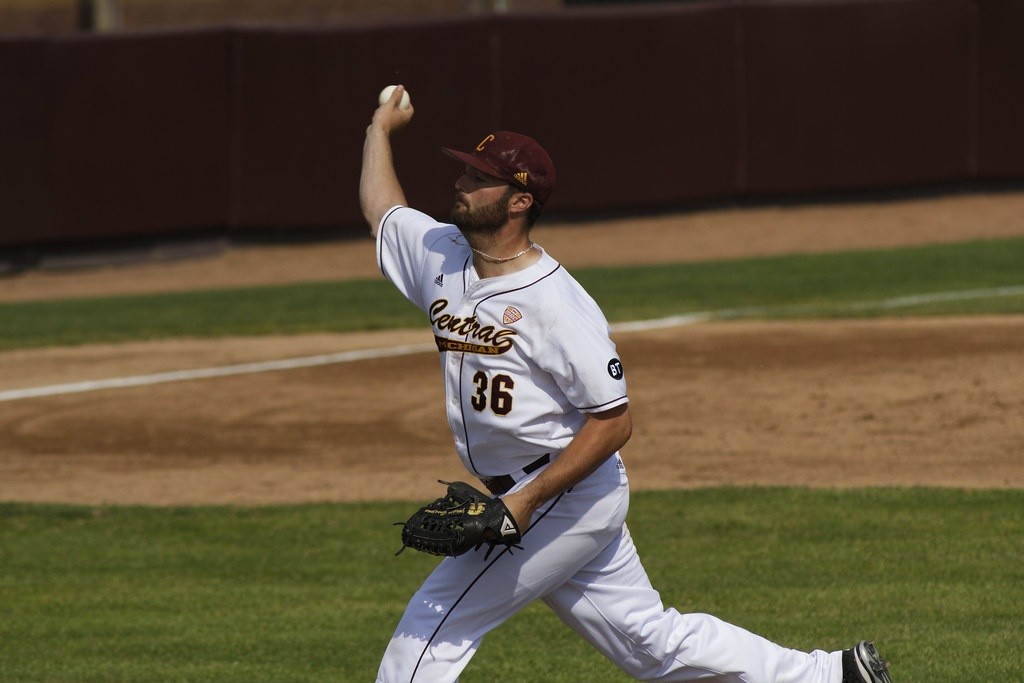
[360,85,891,683]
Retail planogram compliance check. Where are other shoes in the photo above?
[843,640,893,683]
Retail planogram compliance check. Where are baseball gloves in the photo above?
[400,480,522,557]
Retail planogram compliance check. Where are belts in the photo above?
[482,453,550,495]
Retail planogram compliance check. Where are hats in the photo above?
[442,131,555,205]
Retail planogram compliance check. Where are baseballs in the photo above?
[377,85,409,112]
